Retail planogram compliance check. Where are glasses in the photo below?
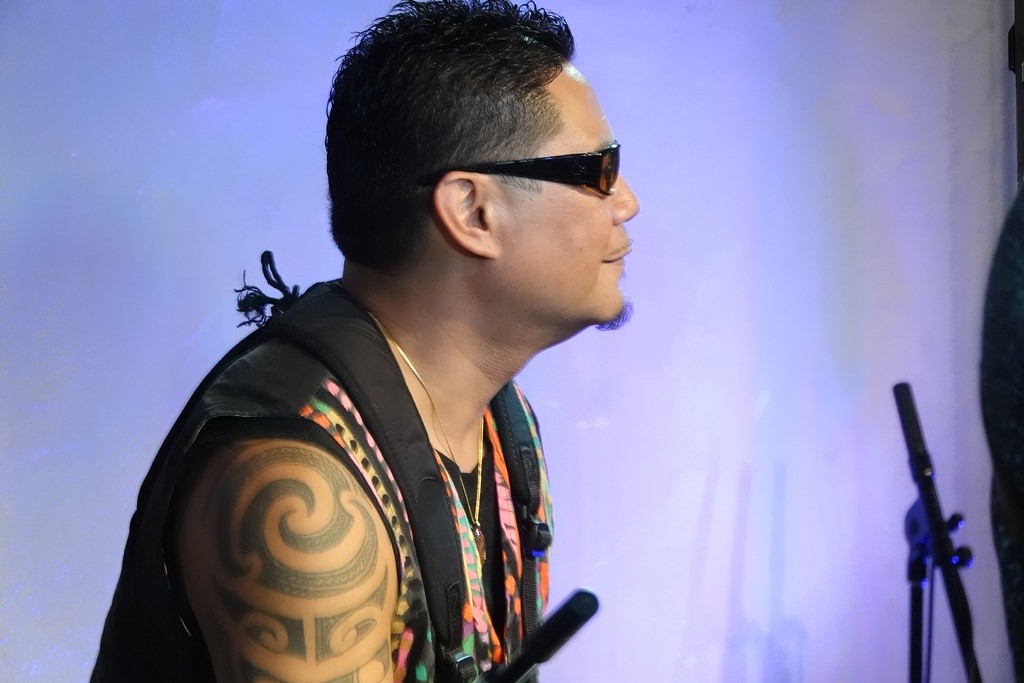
[450,141,624,194]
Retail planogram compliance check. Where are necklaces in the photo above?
[335,280,487,565]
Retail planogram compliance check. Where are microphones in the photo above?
[519,587,598,665]
[891,381,935,483]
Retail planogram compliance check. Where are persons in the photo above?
[87,1,640,683]
